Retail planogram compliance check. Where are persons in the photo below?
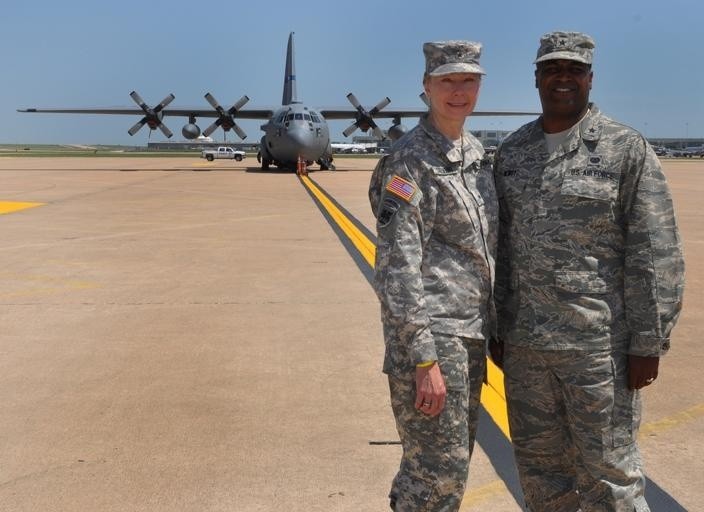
[492,29,686,512]
[368,40,499,511]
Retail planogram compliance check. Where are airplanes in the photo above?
[19,30,570,173]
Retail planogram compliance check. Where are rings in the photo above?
[423,400,431,407]
[646,378,654,383]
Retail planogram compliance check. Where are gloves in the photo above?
[629,355,659,389]
[488,339,503,370]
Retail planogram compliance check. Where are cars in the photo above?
[647,139,703,159]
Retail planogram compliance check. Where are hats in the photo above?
[422,40,487,77]
[533,31,595,68]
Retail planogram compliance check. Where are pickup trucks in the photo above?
[200,143,245,162]
